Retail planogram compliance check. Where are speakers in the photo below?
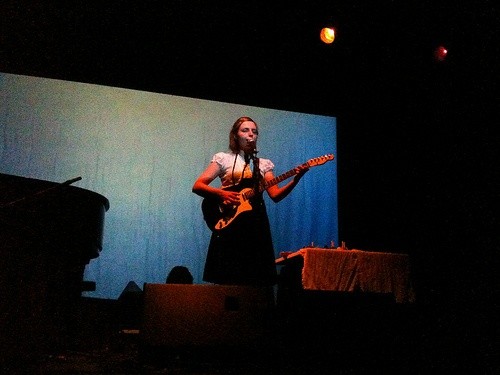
[137,283,262,368]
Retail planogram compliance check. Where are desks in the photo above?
[275,247,418,302]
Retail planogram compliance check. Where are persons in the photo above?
[194,116,311,284]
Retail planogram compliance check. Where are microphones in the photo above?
[248,140,257,152]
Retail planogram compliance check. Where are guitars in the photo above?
[201,154,334,236]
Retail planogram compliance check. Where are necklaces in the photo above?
[231,153,252,188]
